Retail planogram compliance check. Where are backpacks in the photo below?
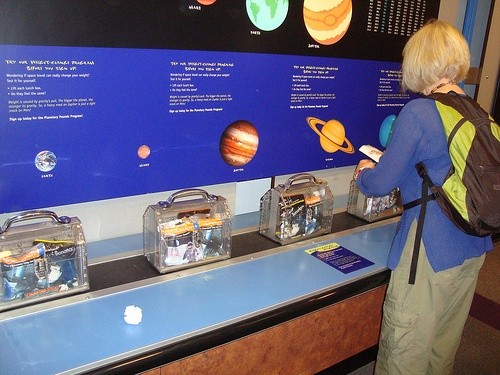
[414,91,500,237]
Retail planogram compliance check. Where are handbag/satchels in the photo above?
[142,189,233,275]
[0,210,90,314]
[258,172,334,245]
[346,164,404,224]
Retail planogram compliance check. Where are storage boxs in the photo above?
[0,211,91,313]
[142,188,233,274]
[347,180,403,224]
[258,172,335,246]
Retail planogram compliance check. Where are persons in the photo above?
[354,15,500,375]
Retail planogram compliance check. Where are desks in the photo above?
[0,202,420,375]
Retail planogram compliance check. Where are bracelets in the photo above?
[356,167,368,178]
